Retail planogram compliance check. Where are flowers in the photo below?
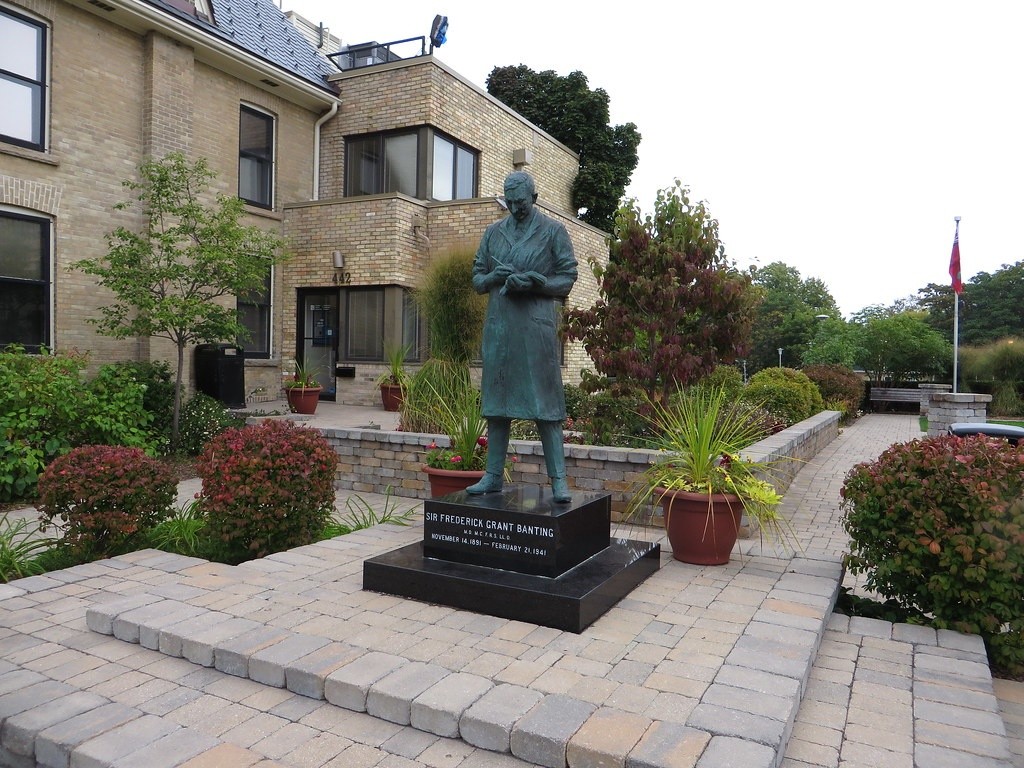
[390,376,518,483]
[612,372,822,569]
[280,352,332,401]
[371,335,415,405]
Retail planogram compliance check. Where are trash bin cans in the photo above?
[946,422,1024,448]
[194,342,247,409]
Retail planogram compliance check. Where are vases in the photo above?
[654,486,744,565]
[378,383,406,412]
[281,386,323,415]
[422,462,486,498]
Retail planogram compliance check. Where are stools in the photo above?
[948,422,1024,448]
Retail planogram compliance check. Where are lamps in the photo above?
[333,251,344,269]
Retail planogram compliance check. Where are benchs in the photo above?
[868,387,921,415]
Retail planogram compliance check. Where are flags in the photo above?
[949,226,962,294]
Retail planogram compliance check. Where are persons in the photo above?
[466,171,579,502]
